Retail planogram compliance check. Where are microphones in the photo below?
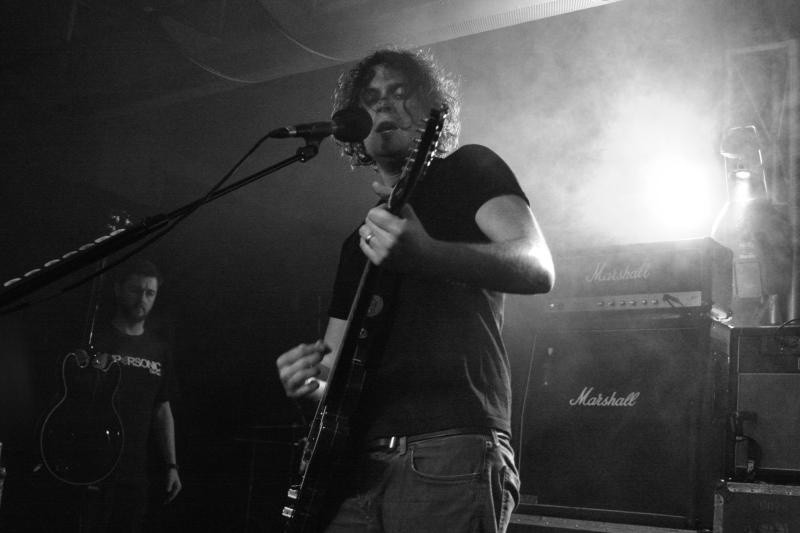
[270,106,373,143]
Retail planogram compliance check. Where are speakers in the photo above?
[515,313,734,531]
[729,325,800,485]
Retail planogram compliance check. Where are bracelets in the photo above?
[166,463,179,470]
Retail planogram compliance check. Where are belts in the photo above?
[365,423,515,453]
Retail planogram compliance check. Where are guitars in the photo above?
[38,207,131,487]
[269,103,451,533]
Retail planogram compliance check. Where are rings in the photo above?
[365,233,375,244]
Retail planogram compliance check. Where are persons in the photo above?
[76,260,181,532]
[276,50,555,533]
